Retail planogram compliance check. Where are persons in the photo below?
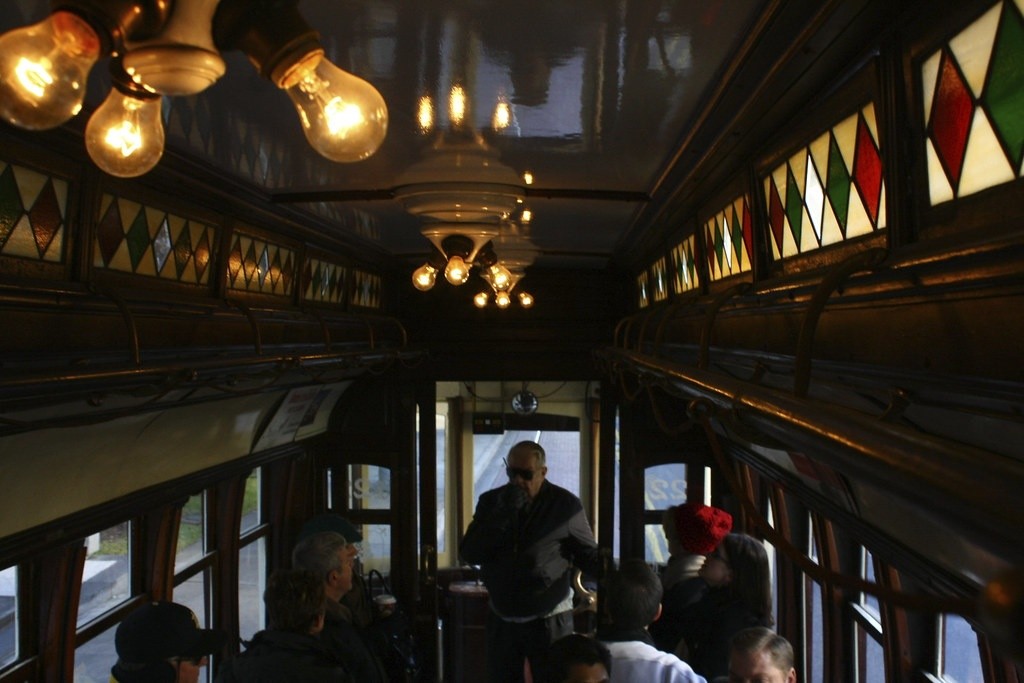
[549,634,611,683]
[593,560,709,683]
[232,570,354,683]
[675,534,775,683]
[457,441,599,683]
[636,501,732,653]
[293,531,388,683]
[729,627,797,683]
[107,602,224,683]
[297,515,383,683]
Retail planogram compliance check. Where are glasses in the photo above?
[710,552,729,565]
[507,467,538,480]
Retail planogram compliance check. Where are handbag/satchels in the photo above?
[358,570,422,683]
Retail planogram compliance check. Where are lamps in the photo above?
[412,223,511,293]
[475,271,533,308]
[0,2,387,178]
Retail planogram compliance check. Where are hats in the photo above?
[674,502,733,555]
[115,601,229,662]
[303,515,363,543]
